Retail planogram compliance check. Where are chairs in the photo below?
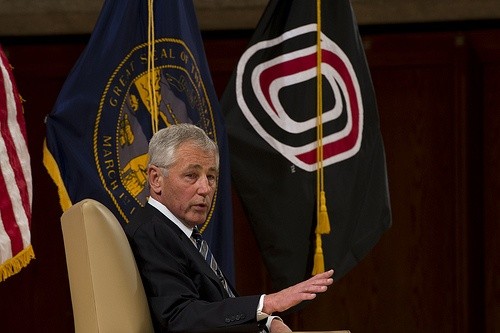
[60,199,350,333]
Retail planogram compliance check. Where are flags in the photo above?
[43,0,236,284]
[0,48,35,282]
[218,0,393,315]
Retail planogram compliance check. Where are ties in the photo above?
[191,226,235,298]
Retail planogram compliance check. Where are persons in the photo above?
[124,123,334,333]
